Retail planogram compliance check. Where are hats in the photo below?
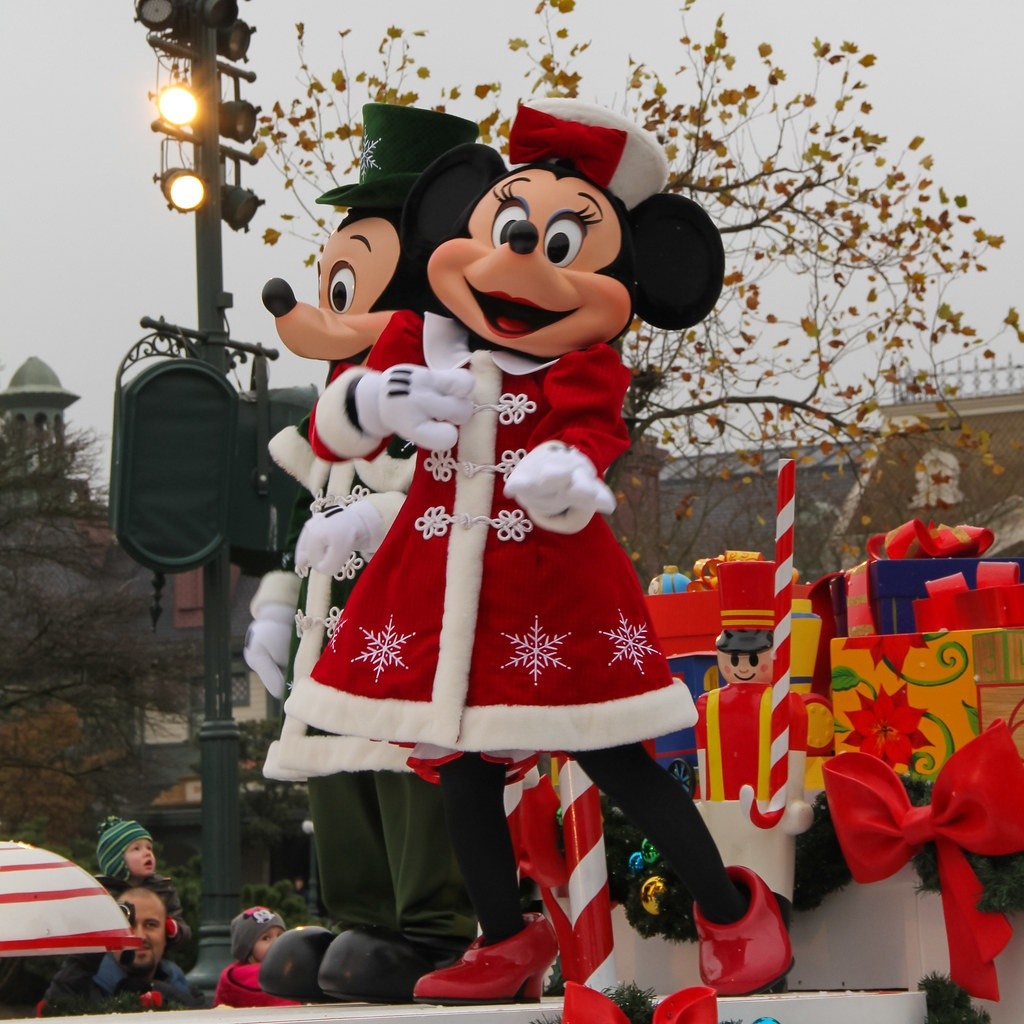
[96,815,153,881]
[231,906,286,963]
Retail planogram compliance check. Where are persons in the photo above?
[213,907,303,1008]
[0,814,209,1017]
[690,556,813,990]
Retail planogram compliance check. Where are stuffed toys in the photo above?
[284,98,793,1004]
[244,102,478,999]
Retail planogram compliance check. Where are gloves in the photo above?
[165,916,178,938]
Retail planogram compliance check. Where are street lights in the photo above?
[133,0,268,1000]
